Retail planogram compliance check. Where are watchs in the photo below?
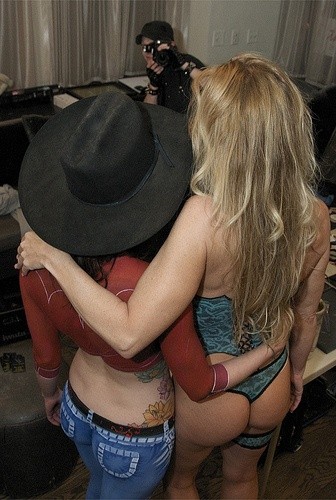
[184,61,196,75]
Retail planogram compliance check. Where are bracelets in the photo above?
[146,84,159,96]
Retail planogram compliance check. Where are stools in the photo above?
[0,339,79,498]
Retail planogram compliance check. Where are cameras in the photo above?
[143,40,170,67]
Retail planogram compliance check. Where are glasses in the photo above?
[142,41,165,53]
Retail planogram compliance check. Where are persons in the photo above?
[18,89,287,500]
[15,52,331,500]
[135,20,207,113]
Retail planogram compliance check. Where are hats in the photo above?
[135,21,174,45]
[18,92,195,256]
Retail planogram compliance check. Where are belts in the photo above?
[66,379,174,439]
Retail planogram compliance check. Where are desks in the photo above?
[259,228,336,500]
